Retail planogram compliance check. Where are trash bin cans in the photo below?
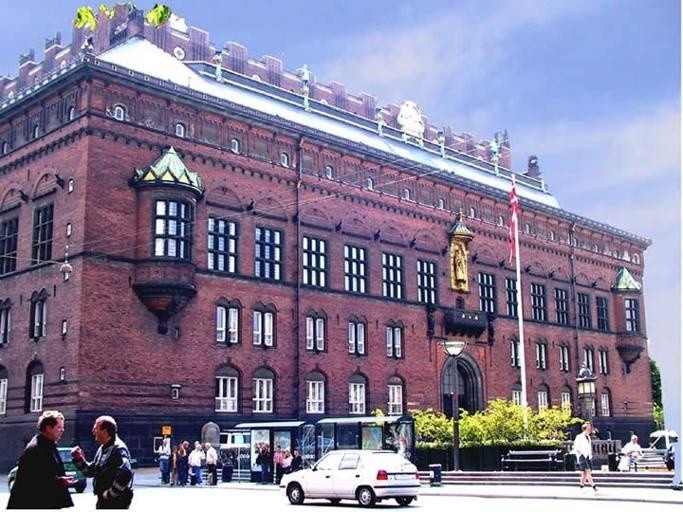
[608,452,619,471]
[564,452,577,471]
[221,463,234,482]
[428,464,442,488]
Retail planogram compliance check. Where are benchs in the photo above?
[500,449,564,471]
[622,449,669,472]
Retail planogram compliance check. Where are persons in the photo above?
[4,407,77,510]
[572,422,600,491]
[71,413,135,509]
[617,432,645,472]
[153,436,303,487]
[664,430,682,489]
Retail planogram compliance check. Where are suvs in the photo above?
[218,429,250,451]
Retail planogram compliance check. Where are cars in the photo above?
[302,436,334,451]
[281,448,422,508]
[9,447,90,493]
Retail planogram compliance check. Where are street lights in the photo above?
[436,338,469,472]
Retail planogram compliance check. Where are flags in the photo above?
[506,181,521,266]
